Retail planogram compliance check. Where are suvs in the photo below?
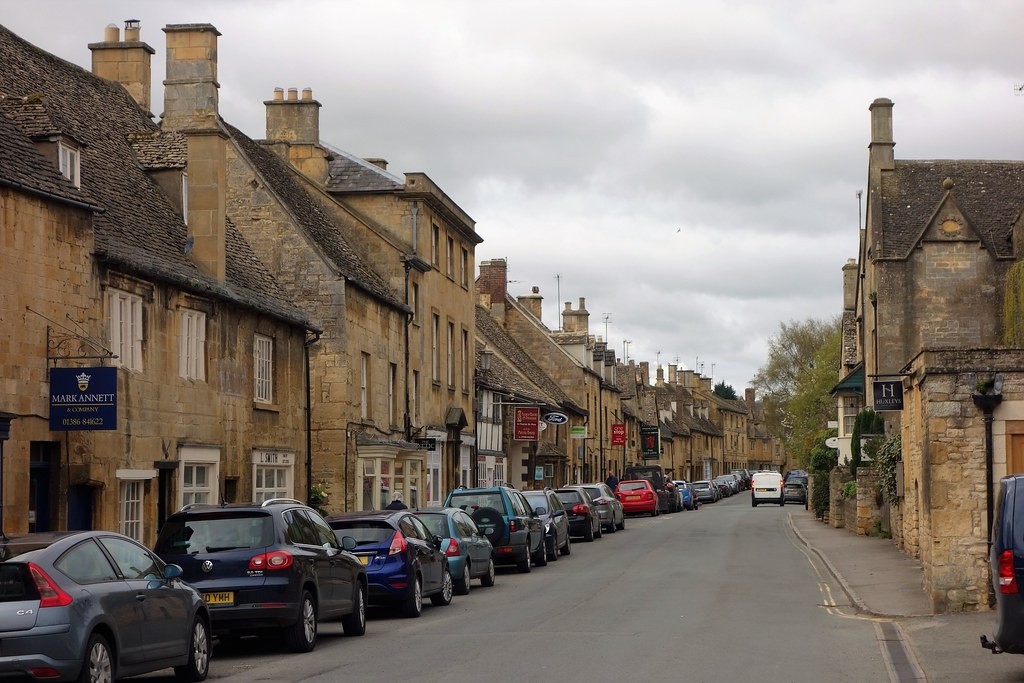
[152,497,367,653]
[564,482,625,534]
[731,470,753,489]
[980,476,1022,657]
[445,482,548,573]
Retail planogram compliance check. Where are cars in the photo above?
[614,480,659,517]
[324,510,454,617]
[519,487,571,561]
[749,470,778,476]
[664,474,745,512]
[409,508,495,596]
[784,470,808,505]
[552,486,601,542]
[0,528,214,683]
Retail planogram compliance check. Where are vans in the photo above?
[751,472,785,507]
[624,464,669,514]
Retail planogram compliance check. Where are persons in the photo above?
[606,470,619,492]
[386,492,408,510]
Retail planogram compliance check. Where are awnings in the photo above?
[829,361,864,395]
